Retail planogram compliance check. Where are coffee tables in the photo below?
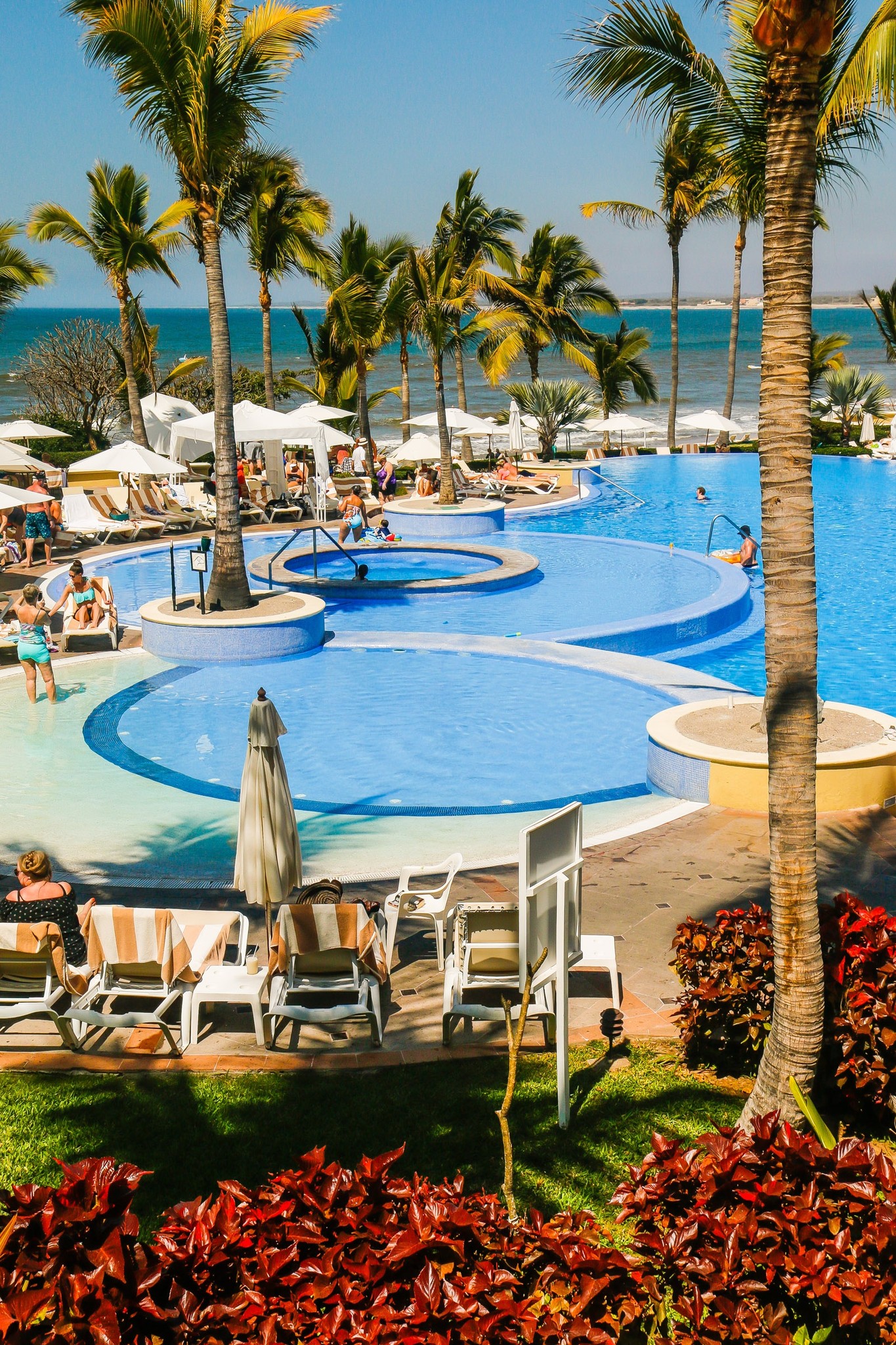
[570,935,621,1009]
[190,965,273,1046]
[11,620,53,645]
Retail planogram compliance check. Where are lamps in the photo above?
[600,1008,624,1064]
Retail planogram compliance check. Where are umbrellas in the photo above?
[0,392,838,516]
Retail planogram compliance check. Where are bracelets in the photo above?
[49,518,54,521]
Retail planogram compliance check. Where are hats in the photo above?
[738,525,752,535]
[358,437,368,445]
[295,879,344,905]
[32,473,48,480]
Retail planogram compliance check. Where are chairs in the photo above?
[521,452,538,461]
[735,434,751,442]
[681,444,700,453]
[866,439,892,457]
[261,903,386,1048]
[585,448,606,459]
[451,463,505,502]
[0,449,378,564]
[619,448,638,456]
[710,435,736,444]
[452,460,560,494]
[656,447,670,455]
[56,906,251,1056]
[385,853,464,975]
[0,903,125,1048]
[412,465,439,499]
[440,901,557,1046]
[505,457,516,465]
[60,577,119,653]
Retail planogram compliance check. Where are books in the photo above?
[388,890,425,911]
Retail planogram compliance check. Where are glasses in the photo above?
[13,868,22,876]
[69,574,78,578]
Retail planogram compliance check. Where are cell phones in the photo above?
[37,591,43,602]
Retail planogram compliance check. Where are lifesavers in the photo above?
[711,549,741,563]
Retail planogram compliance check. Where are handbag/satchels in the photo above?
[283,498,308,517]
[109,508,129,521]
[265,492,288,511]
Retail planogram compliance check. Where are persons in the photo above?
[337,485,370,544]
[47,492,64,538]
[21,473,60,568]
[737,525,759,569]
[12,584,56,702]
[0,507,34,563]
[375,520,395,541]
[1,850,96,967]
[48,560,112,630]
[352,565,369,580]
[295,879,380,917]
